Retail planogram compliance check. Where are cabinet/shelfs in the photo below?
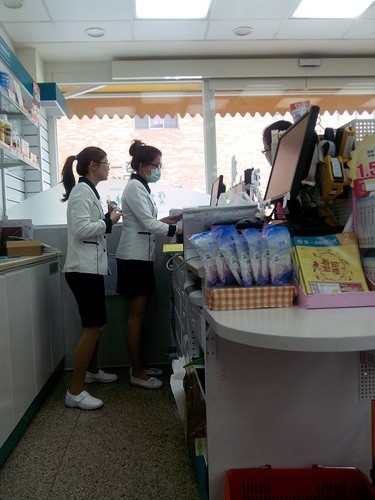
[0,86,40,171]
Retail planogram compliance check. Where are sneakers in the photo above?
[84,368,118,384]
[65,388,104,410]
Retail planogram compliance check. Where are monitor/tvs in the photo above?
[263,104,320,227]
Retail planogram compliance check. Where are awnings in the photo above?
[60,78,375,119]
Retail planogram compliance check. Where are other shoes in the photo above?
[129,374,163,389]
[128,367,165,376]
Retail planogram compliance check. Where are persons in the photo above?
[114,140,182,388]
[261,120,292,166]
[61,147,122,411]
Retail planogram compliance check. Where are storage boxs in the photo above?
[6,238,42,255]
[225,464,375,500]
[203,118,375,310]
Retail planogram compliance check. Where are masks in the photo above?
[265,153,272,165]
[140,164,161,183]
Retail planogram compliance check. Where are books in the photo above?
[290,232,370,295]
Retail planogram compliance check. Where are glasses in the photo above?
[262,148,271,154]
[146,161,163,171]
[95,161,110,167]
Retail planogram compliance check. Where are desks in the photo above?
[205,305,375,353]
